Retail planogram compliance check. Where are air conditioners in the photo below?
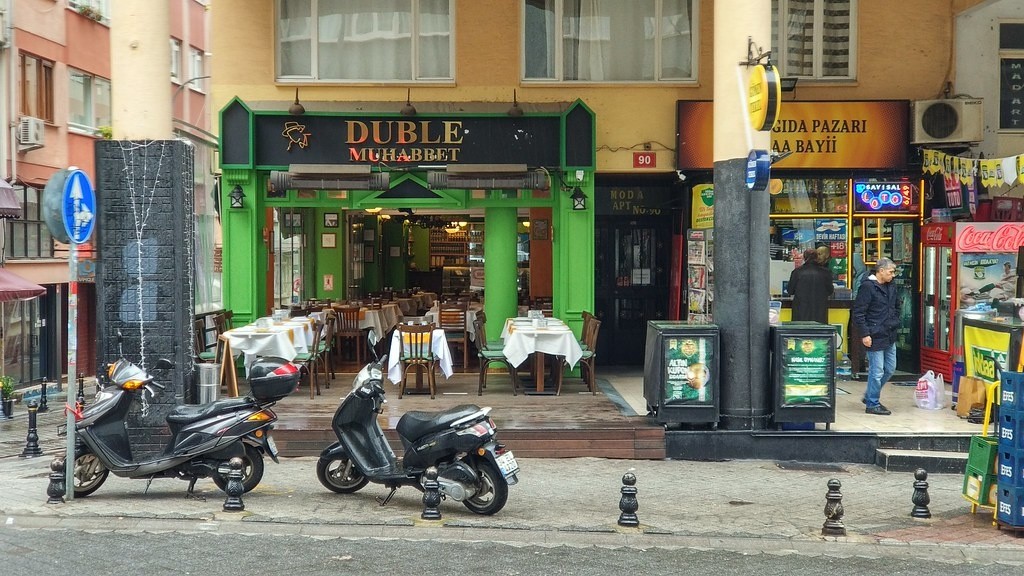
[20,116,45,145]
[908,98,984,144]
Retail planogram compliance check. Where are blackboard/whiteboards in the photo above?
[214,335,239,398]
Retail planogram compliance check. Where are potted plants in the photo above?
[0,376,17,419]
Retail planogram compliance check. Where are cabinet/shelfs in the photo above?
[407,241,415,257]
[429,228,484,288]
[863,218,892,264]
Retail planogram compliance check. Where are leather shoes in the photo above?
[865,407,891,415]
[862,399,888,409]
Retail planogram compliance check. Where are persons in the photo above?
[965,263,1016,303]
[787,249,835,323]
[853,257,901,415]
[816,246,835,272]
[852,253,868,298]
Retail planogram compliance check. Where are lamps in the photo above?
[289,88,305,114]
[780,77,798,92]
[399,87,415,116]
[570,186,588,210]
[507,88,523,116]
[227,185,246,209]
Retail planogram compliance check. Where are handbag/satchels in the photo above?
[956,375,986,417]
[914,370,945,410]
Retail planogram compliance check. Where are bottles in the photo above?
[430,228,484,254]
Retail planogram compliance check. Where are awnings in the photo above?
[0,177,21,216]
[0,267,47,301]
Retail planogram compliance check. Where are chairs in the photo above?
[194,295,471,400]
[473,310,519,396]
[550,311,601,397]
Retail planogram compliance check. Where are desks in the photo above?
[500,317,583,396]
[223,292,484,395]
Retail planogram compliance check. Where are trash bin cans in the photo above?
[193,363,221,405]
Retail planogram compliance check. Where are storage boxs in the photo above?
[963,370,1024,526]
[248,356,301,400]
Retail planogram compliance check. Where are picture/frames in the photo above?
[364,229,401,262]
[532,219,548,240]
[284,213,338,248]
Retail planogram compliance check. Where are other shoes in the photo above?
[851,373,861,381]
[860,365,866,372]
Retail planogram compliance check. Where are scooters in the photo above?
[315,330,521,516]
[62,325,301,499]
